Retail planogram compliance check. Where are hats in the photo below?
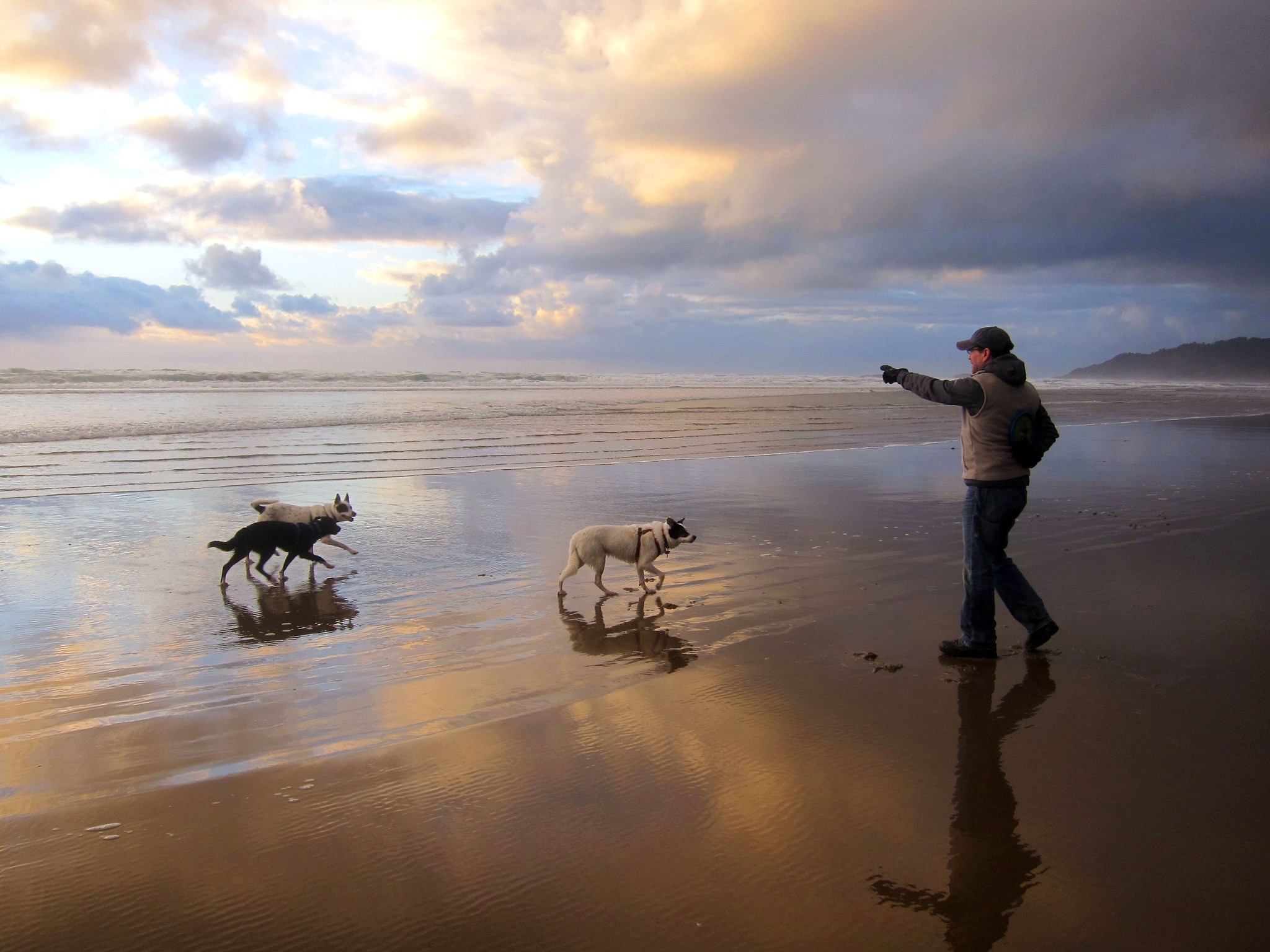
[956,326,1014,351]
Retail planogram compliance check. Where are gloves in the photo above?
[880,364,905,384]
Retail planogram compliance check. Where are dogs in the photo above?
[557,517,697,596]
[206,516,341,590]
[220,562,360,648]
[250,493,360,555]
[558,593,699,674]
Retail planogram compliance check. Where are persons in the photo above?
[880,327,1059,660]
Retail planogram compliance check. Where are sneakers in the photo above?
[939,638,996,661]
[1025,623,1060,651]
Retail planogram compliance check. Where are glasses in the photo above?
[967,349,977,354]
